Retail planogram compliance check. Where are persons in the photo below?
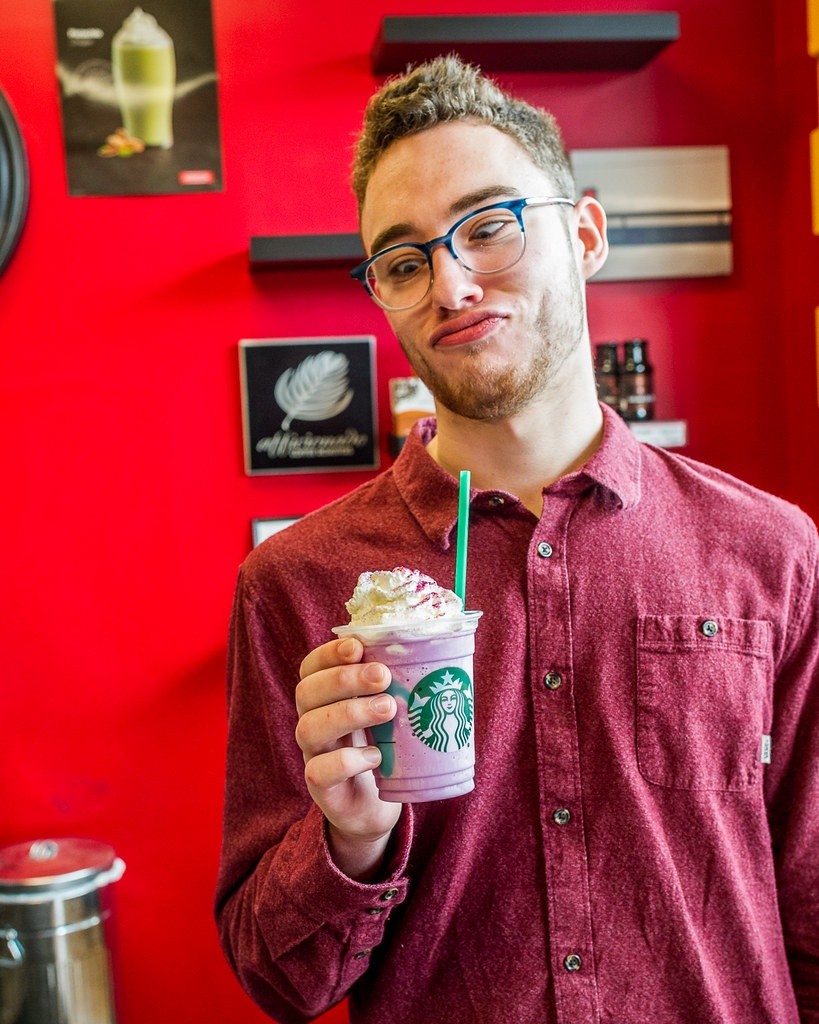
[211,52,819,1022]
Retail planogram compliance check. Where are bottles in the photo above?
[624,342,654,424]
[592,343,620,414]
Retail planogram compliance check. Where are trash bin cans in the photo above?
[0,836,117,1024]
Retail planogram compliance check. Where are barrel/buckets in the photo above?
[0,841,127,1024]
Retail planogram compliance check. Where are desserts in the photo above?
[341,568,467,644]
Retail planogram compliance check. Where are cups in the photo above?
[337,612,481,802]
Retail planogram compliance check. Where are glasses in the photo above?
[349,197,575,311]
